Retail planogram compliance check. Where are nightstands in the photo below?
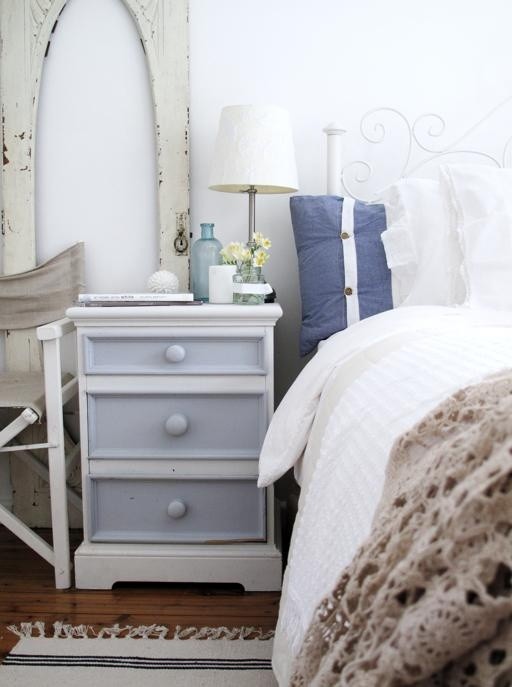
[67,302,286,597]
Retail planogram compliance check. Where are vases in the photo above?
[233,282,271,307]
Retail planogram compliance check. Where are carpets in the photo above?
[0,618,280,687]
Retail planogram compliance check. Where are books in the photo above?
[75,291,197,308]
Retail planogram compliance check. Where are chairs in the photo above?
[0,242,84,593]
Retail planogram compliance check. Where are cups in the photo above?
[209,264,236,304]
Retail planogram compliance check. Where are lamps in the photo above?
[206,101,298,280]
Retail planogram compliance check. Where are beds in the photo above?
[288,92,512,687]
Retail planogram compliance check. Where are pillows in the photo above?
[289,164,511,359]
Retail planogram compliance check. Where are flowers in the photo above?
[217,232,272,285]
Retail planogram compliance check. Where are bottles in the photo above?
[191,223,223,301]
